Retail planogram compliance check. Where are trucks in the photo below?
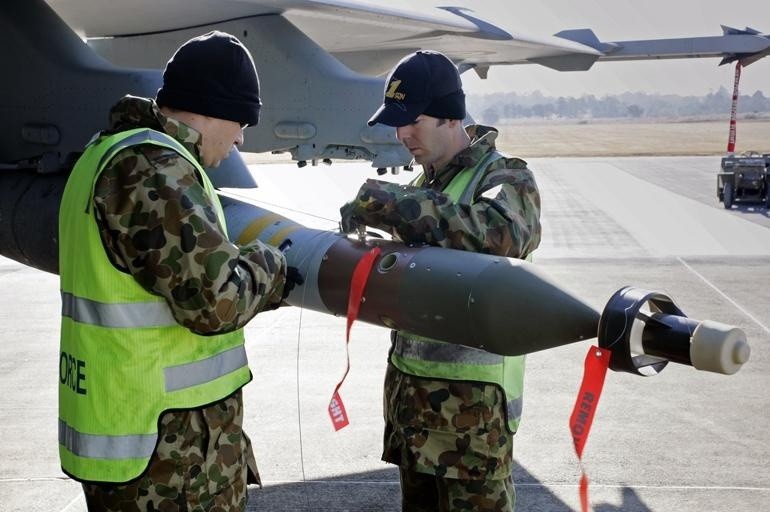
[716,150,769,211]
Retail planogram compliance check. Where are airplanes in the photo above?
[0,0,770,377]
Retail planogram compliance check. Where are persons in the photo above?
[341,48,540,510]
[56,28,306,512]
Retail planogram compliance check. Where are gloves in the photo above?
[340,196,368,236]
[281,265,305,300]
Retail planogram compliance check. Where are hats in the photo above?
[363,49,468,128]
[154,27,265,129]
